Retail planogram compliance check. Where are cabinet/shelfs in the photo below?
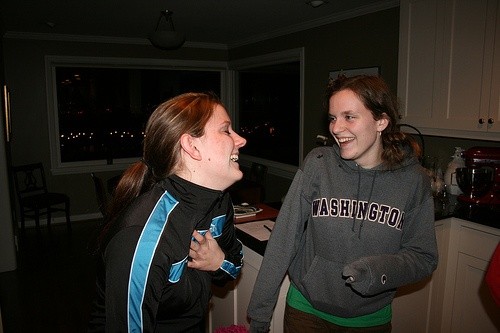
[394,0,500,142]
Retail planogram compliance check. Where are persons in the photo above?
[89,92,248,333]
[247,76,439,333]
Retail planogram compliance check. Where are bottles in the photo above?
[442,147,467,197]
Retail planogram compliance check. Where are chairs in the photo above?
[11,162,73,234]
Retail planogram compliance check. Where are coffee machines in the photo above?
[455,145,500,210]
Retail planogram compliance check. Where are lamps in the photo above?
[152,10,186,49]
